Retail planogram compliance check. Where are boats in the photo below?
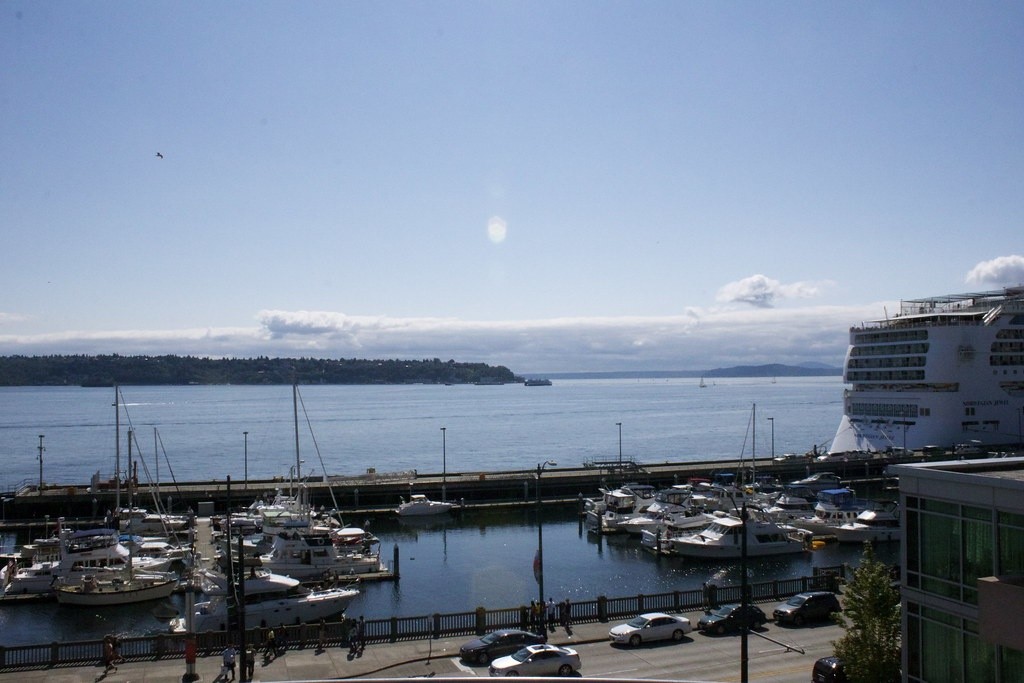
[0,528,194,603]
[524,378,553,386]
[215,496,382,580]
[394,494,455,516]
[582,473,905,557]
[169,572,361,630]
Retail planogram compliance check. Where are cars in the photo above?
[459,629,546,666]
[953,448,982,454]
[697,603,767,635]
[609,612,693,647]
[488,643,582,677]
[811,655,849,683]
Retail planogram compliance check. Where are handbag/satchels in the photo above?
[221,665,229,675]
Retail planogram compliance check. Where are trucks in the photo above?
[956,440,984,450]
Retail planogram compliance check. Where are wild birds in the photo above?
[155,152,163,159]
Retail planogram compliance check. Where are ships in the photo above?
[825,284,1024,455]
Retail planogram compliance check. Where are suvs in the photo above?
[817,439,946,463]
[773,591,842,629]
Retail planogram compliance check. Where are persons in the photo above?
[262,623,289,658]
[245,643,256,680]
[698,582,709,610]
[222,644,236,680]
[344,616,366,654]
[531,597,572,631]
[103,636,126,673]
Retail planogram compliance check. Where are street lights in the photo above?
[290,460,306,496]
[900,412,905,454]
[768,417,774,459]
[440,427,447,502]
[615,422,622,473]
[536,460,562,628]
[243,431,248,490]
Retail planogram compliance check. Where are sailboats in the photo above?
[732,406,780,506]
[699,376,707,387]
[102,382,196,533]
